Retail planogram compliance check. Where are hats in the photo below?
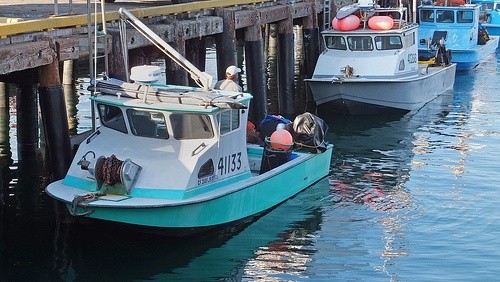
[226,66,241,76]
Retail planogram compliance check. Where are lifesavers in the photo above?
[436,0,465,6]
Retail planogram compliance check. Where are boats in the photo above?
[44,64,334,235]
[466,0,500,36]
[414,0,499,70]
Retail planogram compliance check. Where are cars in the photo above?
[303,1,457,109]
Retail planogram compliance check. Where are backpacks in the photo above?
[247,113,329,153]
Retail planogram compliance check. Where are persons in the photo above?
[478,13,489,41]
[213,65,243,93]
